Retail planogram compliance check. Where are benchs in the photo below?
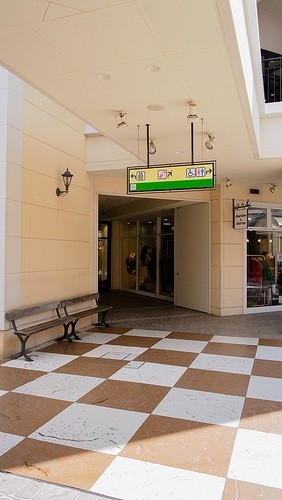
[60,293,112,340]
[5,300,76,361]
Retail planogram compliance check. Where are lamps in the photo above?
[205,131,215,150]
[149,138,156,154]
[269,184,276,194]
[56,167,74,197]
[115,112,126,128]
[226,177,232,188]
[187,101,197,121]
